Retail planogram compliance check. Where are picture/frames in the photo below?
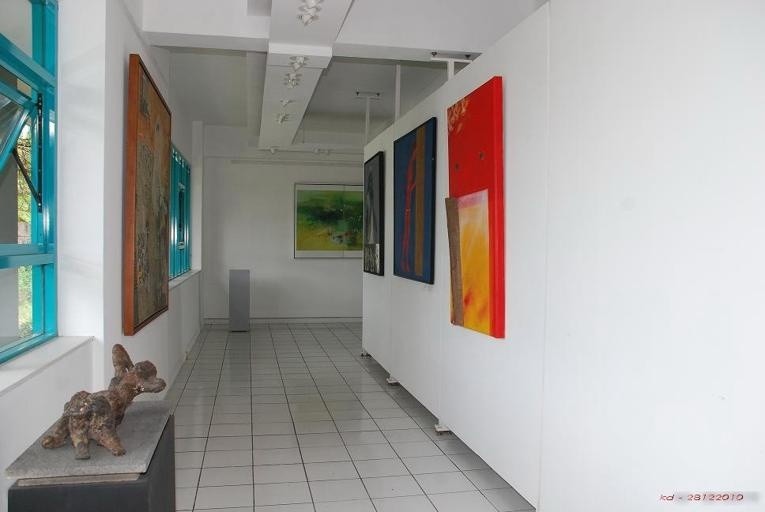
[363,151,383,277]
[122,52,171,335]
[294,183,364,260]
[392,116,437,284]
